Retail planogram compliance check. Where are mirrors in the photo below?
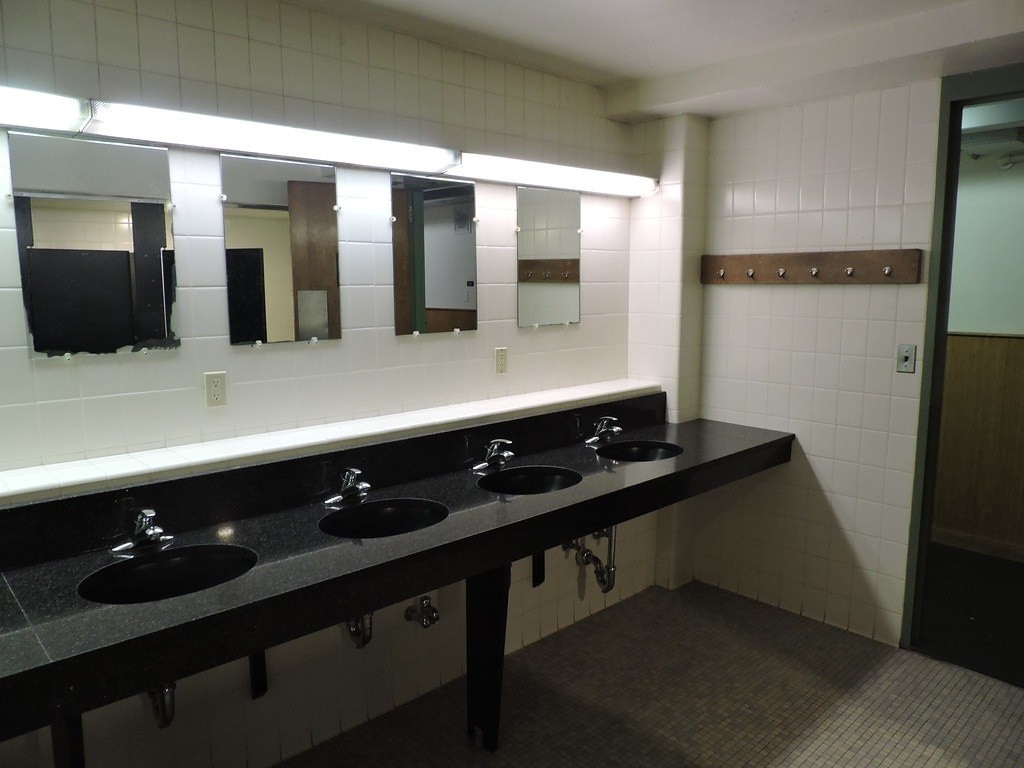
[5,131,184,361]
[218,153,342,346]
[388,172,479,337]
[515,185,582,328]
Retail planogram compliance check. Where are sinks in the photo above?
[476,465,583,495]
[76,544,259,606]
[318,497,450,540]
[596,440,684,462]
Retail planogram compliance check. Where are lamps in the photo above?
[0,86,81,132]
[83,97,456,176]
[445,150,660,197]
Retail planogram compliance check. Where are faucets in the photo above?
[324,468,371,510]
[584,416,623,445]
[110,508,175,552]
[472,438,515,474]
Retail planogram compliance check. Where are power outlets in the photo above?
[896,344,916,373]
[204,371,228,408]
[494,347,508,374]
